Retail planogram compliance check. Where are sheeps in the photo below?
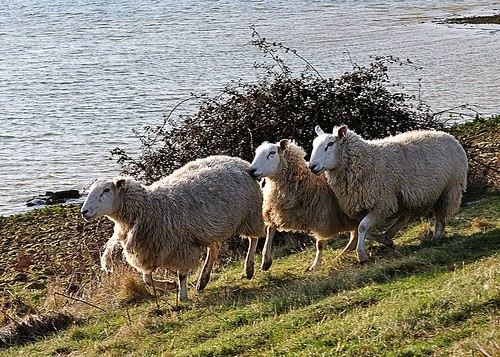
[80,153,265,303]
[246,139,360,273]
[308,125,469,264]
